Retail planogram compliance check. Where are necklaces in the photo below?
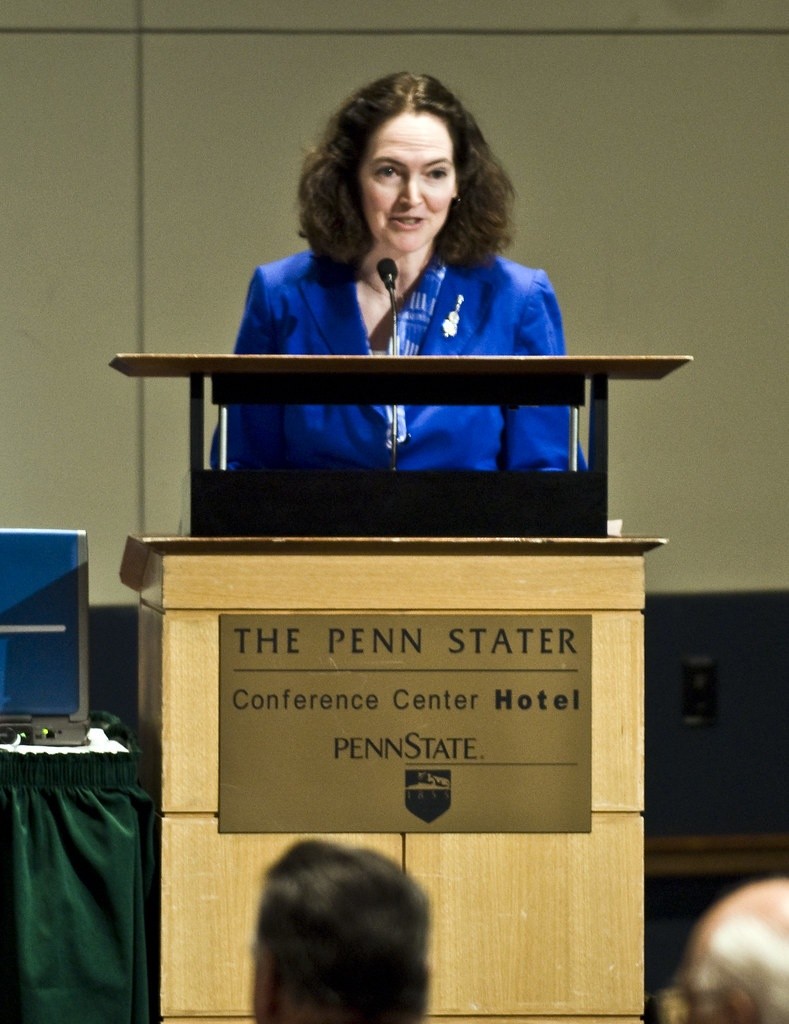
[362,276,403,305]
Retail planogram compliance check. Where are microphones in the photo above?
[376,258,398,471]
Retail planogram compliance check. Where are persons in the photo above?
[211,70,587,472]
[657,879,788,1024]
[253,836,430,1024]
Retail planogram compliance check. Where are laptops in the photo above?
[0,528,91,747]
